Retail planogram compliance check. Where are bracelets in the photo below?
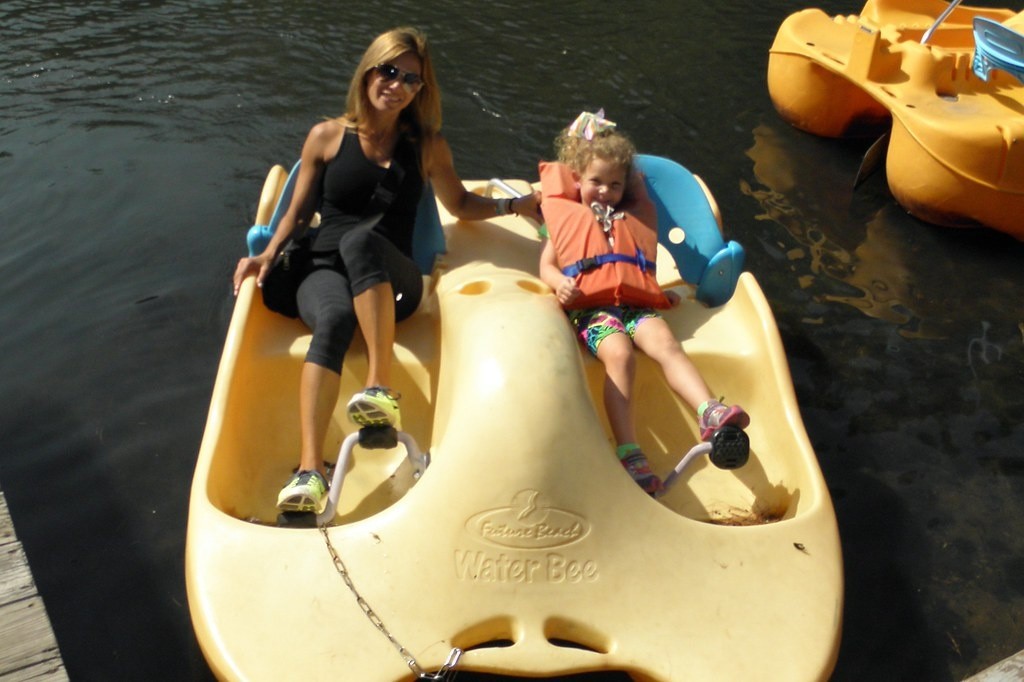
[509,197,517,213]
[496,198,507,214]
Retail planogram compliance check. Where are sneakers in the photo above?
[617,449,660,492]
[346,387,401,428]
[697,397,750,441]
[276,471,329,513]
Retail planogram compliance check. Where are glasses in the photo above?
[377,63,421,84]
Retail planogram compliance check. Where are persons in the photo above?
[539,113,750,498]
[232,28,543,516]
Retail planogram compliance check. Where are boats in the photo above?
[768,0,1024,235]
[184,161,843,682]
